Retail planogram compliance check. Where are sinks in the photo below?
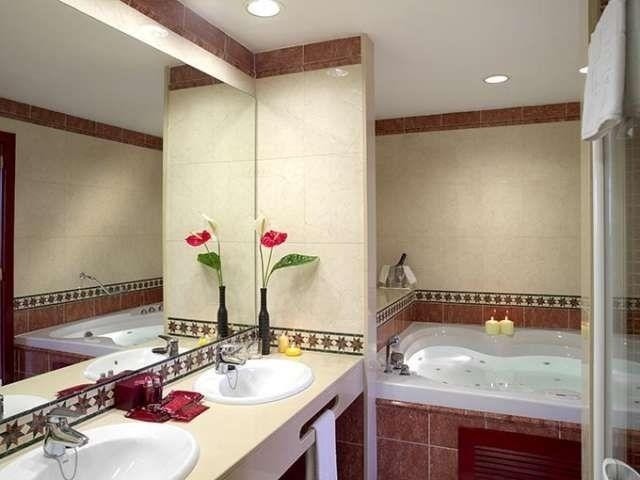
[1,421,198,480]
[84,347,190,379]
[193,357,313,404]
[1,392,49,417]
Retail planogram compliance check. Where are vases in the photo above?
[218,286,227,337]
[258,288,270,355]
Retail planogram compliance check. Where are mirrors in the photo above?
[0,0,256,460]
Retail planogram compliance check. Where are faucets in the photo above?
[216,343,245,372]
[44,407,88,457]
[385,336,399,372]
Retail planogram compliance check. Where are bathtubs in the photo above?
[16,310,163,350]
[376,322,639,430]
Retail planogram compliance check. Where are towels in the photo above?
[306,409,338,480]
[581,0,627,141]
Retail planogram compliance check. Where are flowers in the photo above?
[258,219,320,288]
[185,215,223,286]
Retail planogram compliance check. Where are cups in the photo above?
[286,341,301,356]
[247,338,263,360]
